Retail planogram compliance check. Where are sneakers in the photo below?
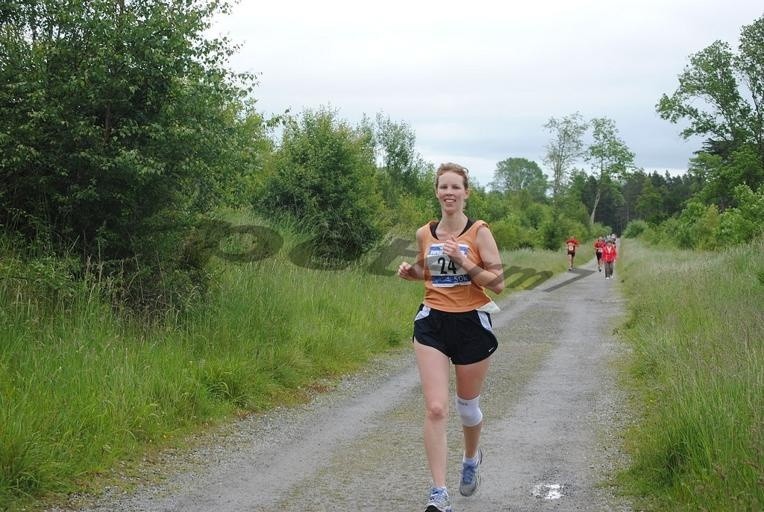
[423,486,451,512]
[568,268,576,271]
[605,275,613,280]
[459,448,483,496]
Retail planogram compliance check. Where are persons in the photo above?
[397,162,504,512]
[591,232,616,280]
[565,234,579,272]
